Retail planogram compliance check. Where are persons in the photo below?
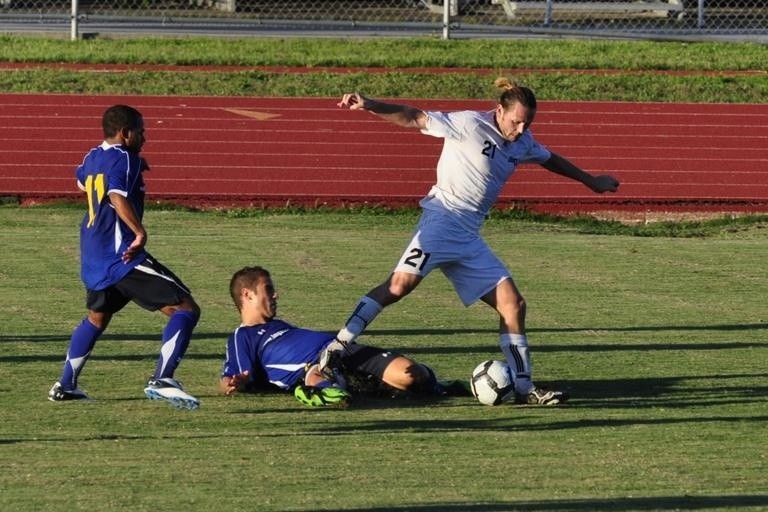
[292,75,623,410]
[219,263,439,407]
[45,103,204,413]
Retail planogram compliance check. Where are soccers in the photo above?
[469,358,515,407]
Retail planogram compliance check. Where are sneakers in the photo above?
[48,382,94,400]
[319,340,341,381]
[515,388,564,404]
[144,376,200,410]
[295,385,350,407]
[440,380,472,396]
